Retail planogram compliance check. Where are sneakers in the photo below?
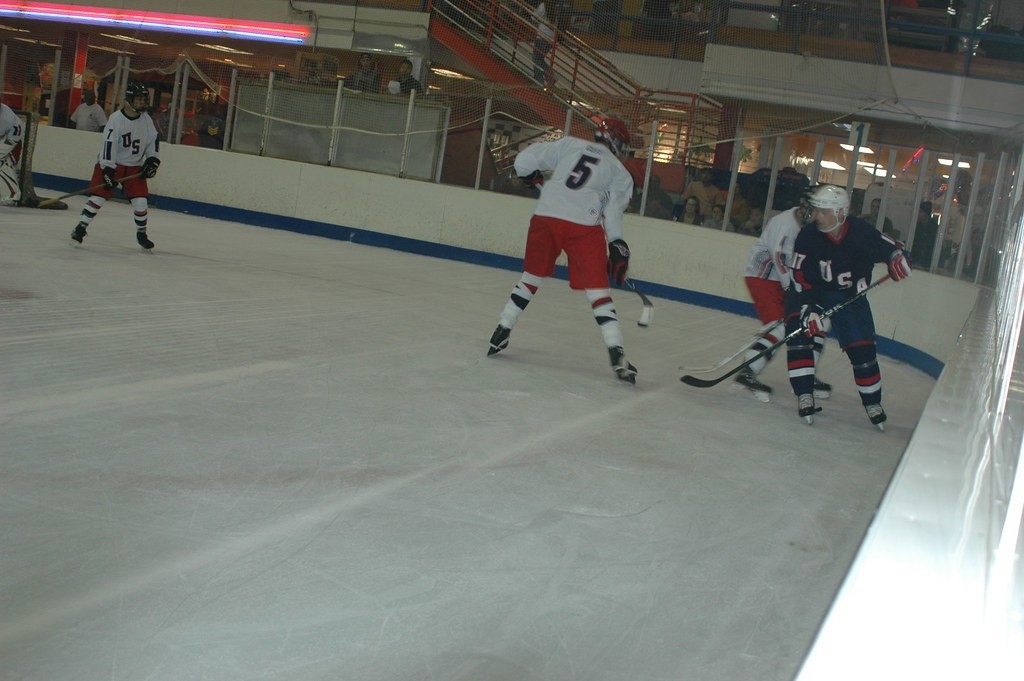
[70,224,87,244]
[862,403,887,431]
[608,346,638,385]
[730,370,773,403]
[796,394,822,424]
[790,376,833,400]
[136,231,154,254]
[485,324,509,357]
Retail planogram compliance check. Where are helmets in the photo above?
[595,118,630,162]
[807,185,851,233]
[799,183,819,208]
[126,79,150,113]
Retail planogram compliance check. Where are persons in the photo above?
[70,80,161,254]
[952,227,986,276]
[0,100,23,205]
[529,0,560,87]
[907,201,944,268]
[486,119,637,384]
[637,166,765,237]
[860,198,893,236]
[345,52,381,93]
[733,184,831,402]
[69,90,108,132]
[780,183,912,429]
[393,60,423,96]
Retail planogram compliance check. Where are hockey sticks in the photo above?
[680,273,892,389]
[536,183,655,328]
[679,317,785,374]
[37,172,143,207]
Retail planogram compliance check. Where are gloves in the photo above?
[518,170,544,190]
[799,303,828,339]
[606,239,630,287]
[138,157,161,179]
[886,248,912,282]
[101,166,118,190]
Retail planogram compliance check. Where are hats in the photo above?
[920,201,933,213]
